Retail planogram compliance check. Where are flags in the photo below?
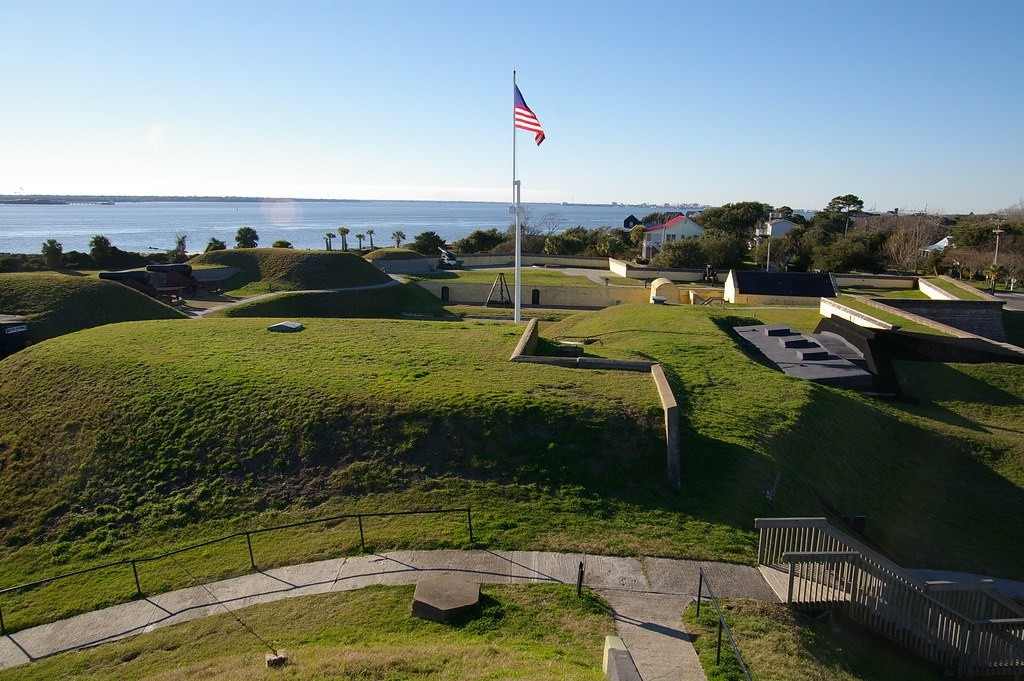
[512,70,546,146]
